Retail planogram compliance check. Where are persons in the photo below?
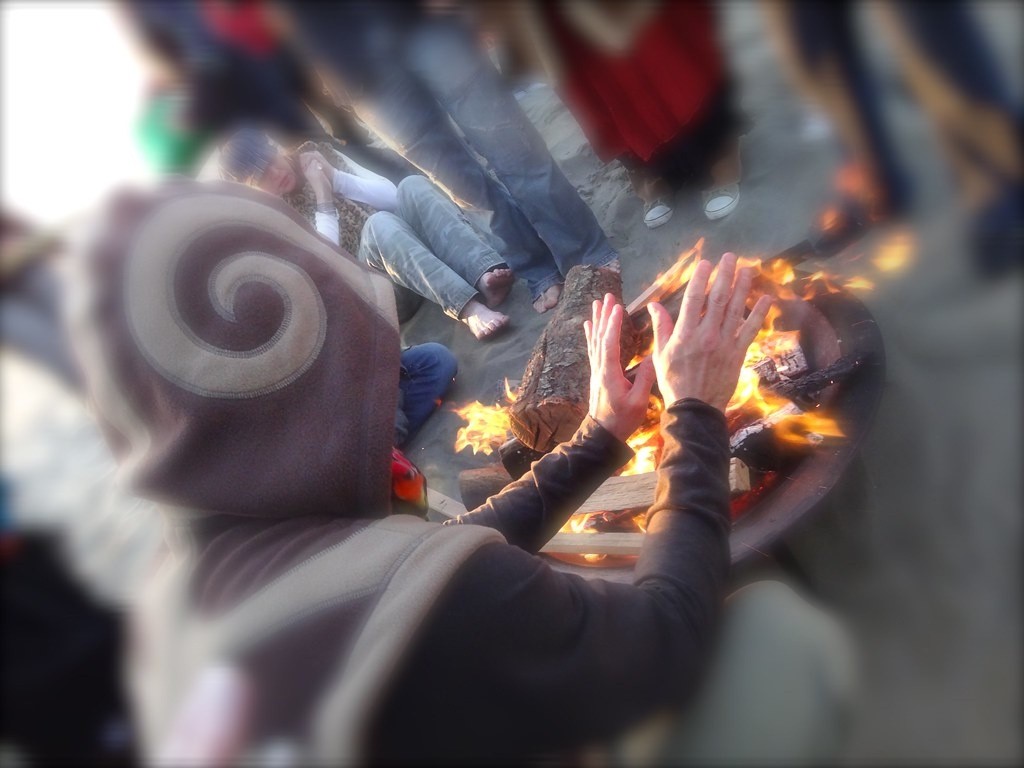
[0,180,858,768]
[106,0,1024,339]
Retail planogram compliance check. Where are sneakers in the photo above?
[704,185,740,220]
[642,201,672,228]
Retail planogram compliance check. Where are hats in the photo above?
[220,130,278,185]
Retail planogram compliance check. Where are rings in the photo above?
[316,166,322,170]
